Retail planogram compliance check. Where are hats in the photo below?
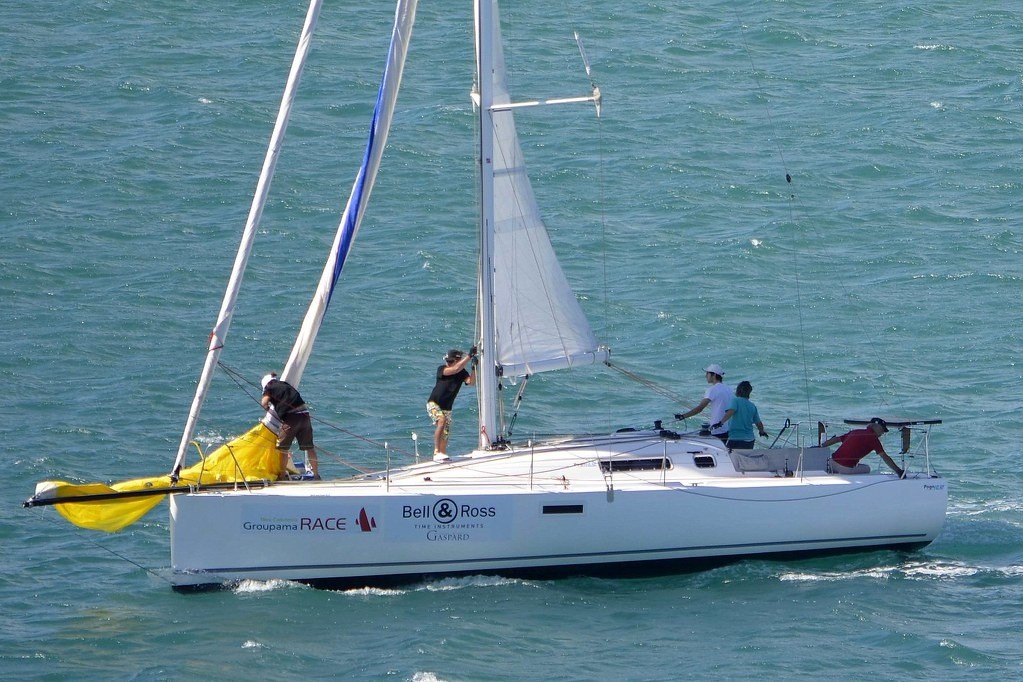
[871,418,889,432]
[702,364,724,378]
[445,350,462,361]
[260,375,276,394]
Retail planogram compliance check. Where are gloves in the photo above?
[471,356,478,370]
[897,469,907,480]
[710,421,723,430]
[468,345,477,359]
[675,415,685,420]
[759,432,769,440]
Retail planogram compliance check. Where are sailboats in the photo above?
[22,0,952,595]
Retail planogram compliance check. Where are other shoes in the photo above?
[314,475,322,481]
[276,474,286,481]
[433,452,449,461]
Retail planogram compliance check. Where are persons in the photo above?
[810,418,907,479]
[261,373,322,481]
[674,365,733,438]
[426,346,478,460]
[708,381,769,449]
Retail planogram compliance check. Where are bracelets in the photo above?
[266,407,269,410]
[821,445,822,447]
